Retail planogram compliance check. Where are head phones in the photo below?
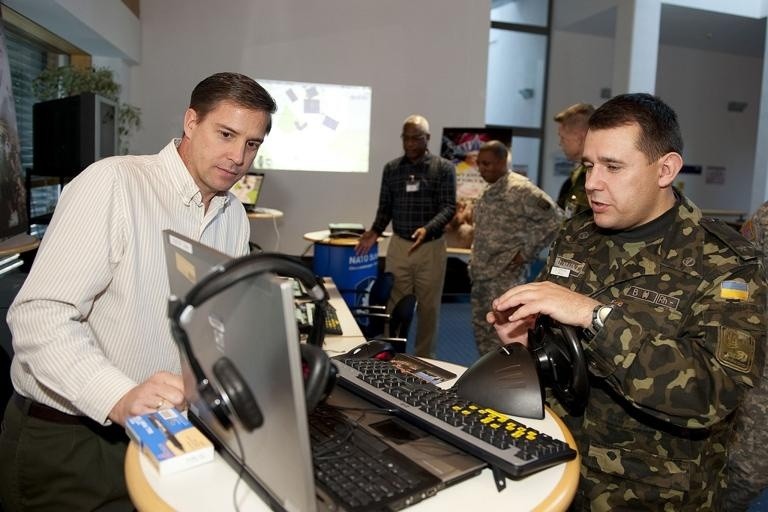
[164,253,338,433]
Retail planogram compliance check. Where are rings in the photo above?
[156,396,166,410]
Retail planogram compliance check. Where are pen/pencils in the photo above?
[148,415,184,452]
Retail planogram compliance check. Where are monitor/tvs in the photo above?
[31,91,119,178]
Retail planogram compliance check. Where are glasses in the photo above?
[399,133,423,142]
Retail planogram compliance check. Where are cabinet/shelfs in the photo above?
[27,169,77,234]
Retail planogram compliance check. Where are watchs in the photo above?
[585,299,616,337]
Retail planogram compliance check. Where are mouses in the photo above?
[347,338,394,363]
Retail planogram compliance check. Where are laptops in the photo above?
[159,227,490,512]
[227,173,264,212]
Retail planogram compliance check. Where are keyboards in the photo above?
[304,300,343,334]
[330,354,578,481]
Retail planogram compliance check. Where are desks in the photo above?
[246,207,285,251]
[304,229,385,329]
[124,354,581,511]
[0,234,40,254]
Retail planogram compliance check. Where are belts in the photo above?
[401,230,447,243]
[13,394,85,425]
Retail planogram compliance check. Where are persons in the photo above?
[356,115,458,358]
[1,71,276,512]
[718,200,767,512]
[467,140,561,360]
[486,93,767,512]
[555,104,596,206]
